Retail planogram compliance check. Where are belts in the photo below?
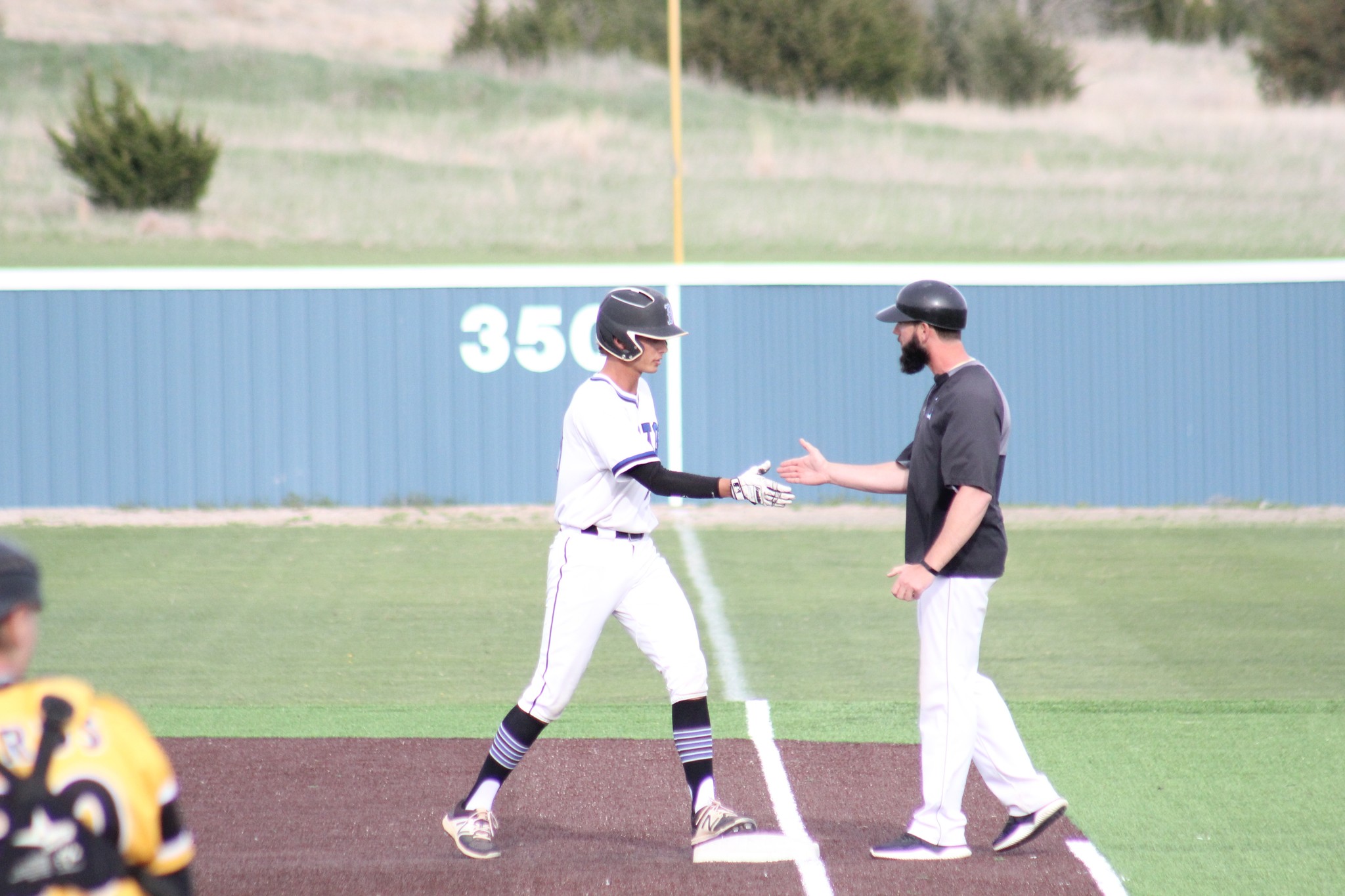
[580,524,643,541]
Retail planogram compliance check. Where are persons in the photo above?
[0,538,198,896]
[439,284,798,862]
[774,278,1071,864]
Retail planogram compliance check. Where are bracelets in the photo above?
[920,559,940,577]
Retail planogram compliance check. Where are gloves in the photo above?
[728,459,794,509]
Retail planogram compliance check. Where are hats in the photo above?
[0,543,43,617]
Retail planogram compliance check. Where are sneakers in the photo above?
[869,836,972,859]
[439,800,501,859]
[993,797,1066,850]
[690,801,756,845]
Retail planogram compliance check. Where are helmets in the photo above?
[595,287,689,362]
[875,278,968,330]
